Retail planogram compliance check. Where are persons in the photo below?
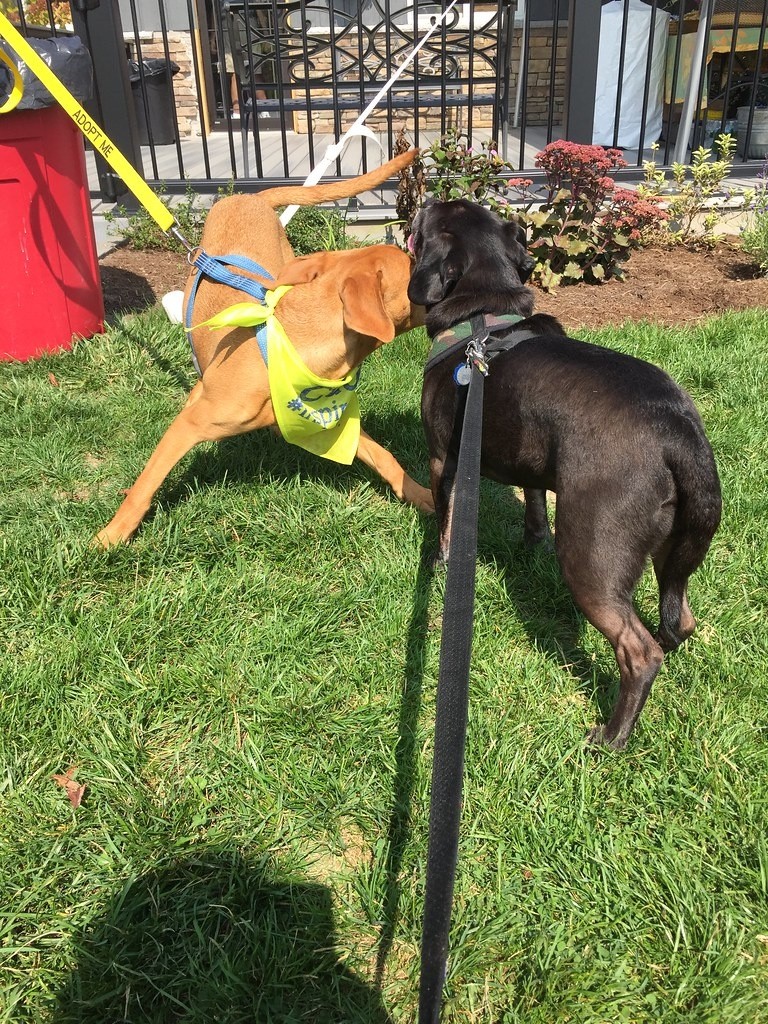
[209,0,271,120]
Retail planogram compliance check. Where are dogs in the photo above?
[88,148,722,743]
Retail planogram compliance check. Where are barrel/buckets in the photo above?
[736,106,768,160]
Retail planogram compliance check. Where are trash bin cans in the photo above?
[128,58,178,144]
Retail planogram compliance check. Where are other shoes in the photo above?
[231,113,240,119]
[259,111,271,118]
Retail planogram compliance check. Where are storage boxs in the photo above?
[689,105,768,159]
